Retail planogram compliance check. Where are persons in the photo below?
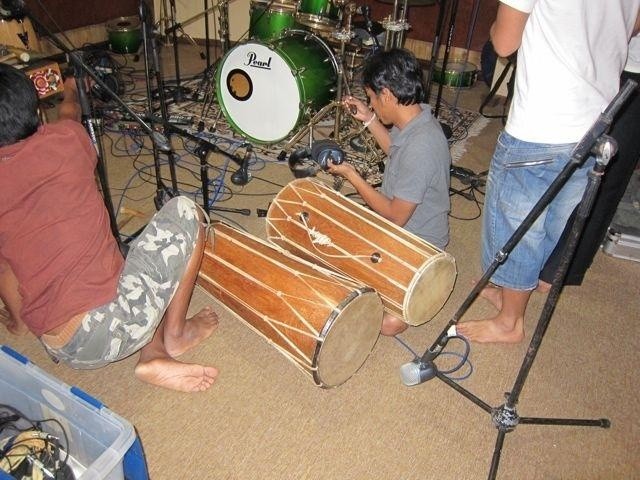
[327,49,451,336]
[0,63,219,392]
[538,34,640,288]
[456,1,639,345]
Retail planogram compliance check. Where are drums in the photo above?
[292,0,346,33]
[215,28,339,145]
[105,14,143,54]
[430,58,478,91]
[249,1,309,41]
[195,220,384,388]
[265,177,456,326]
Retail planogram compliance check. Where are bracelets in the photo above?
[363,113,376,126]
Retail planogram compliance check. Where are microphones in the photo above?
[401,339,447,386]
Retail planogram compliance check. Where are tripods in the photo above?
[423,78,638,480]
[9,0,475,259]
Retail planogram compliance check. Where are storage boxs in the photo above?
[1,344,147,480]
